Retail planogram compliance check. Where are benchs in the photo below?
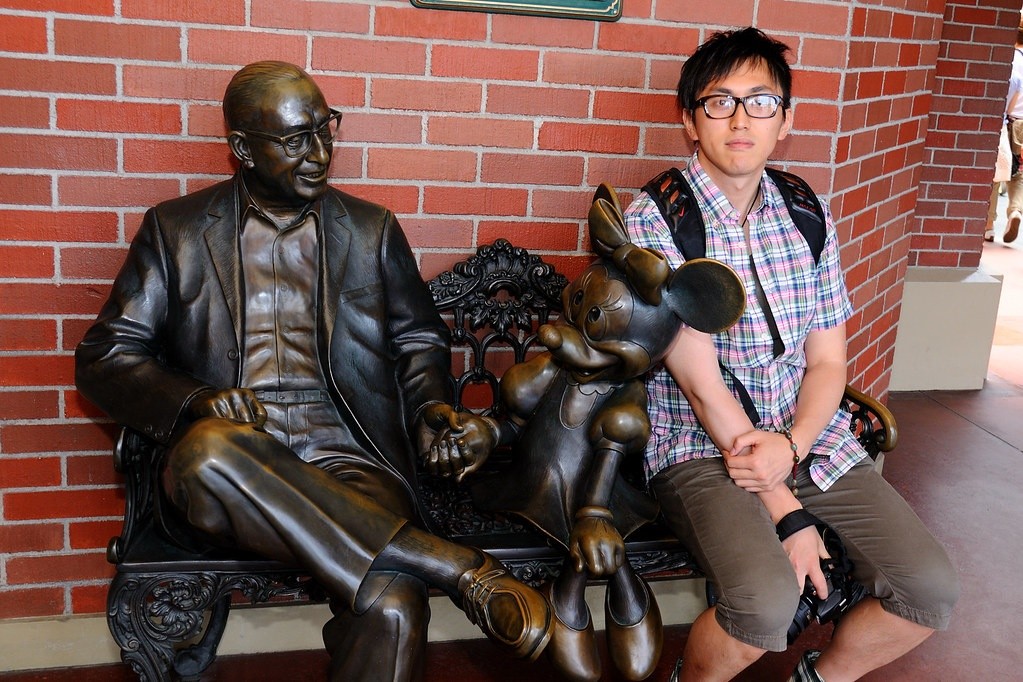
[106,239,897,682]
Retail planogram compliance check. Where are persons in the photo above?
[984,24,1023,243]
[74,60,555,682]
[622,27,959,682]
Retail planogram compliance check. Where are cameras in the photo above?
[785,559,866,645]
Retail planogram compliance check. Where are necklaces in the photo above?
[741,183,760,226]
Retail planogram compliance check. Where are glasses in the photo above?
[689,92,787,120]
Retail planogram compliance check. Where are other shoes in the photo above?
[669,656,683,682]
[786,648,829,682]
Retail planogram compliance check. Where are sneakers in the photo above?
[985,229,995,242]
[1002,211,1022,243]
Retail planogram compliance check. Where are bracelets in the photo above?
[779,429,798,496]
